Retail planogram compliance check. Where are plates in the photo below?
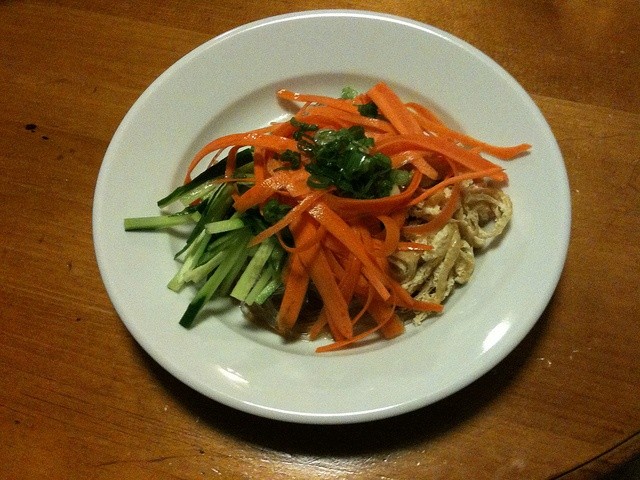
[90,9,572,426]
